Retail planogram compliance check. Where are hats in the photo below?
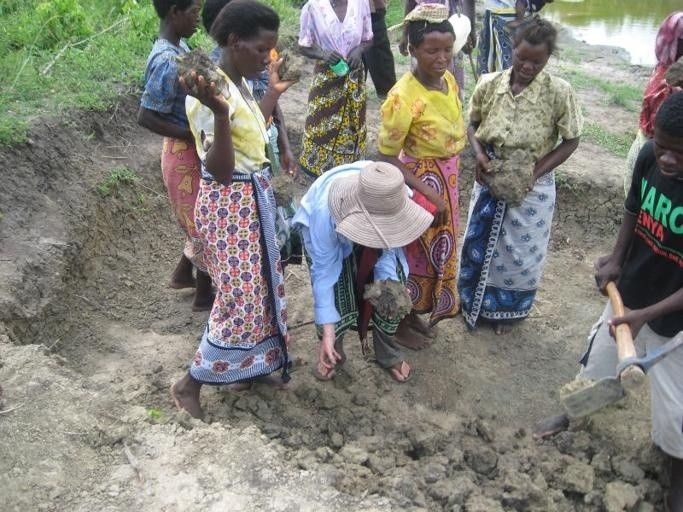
[328,162,434,250]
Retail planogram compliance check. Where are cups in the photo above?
[329,57,350,77]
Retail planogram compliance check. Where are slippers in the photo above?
[388,360,412,383]
[312,355,346,382]
